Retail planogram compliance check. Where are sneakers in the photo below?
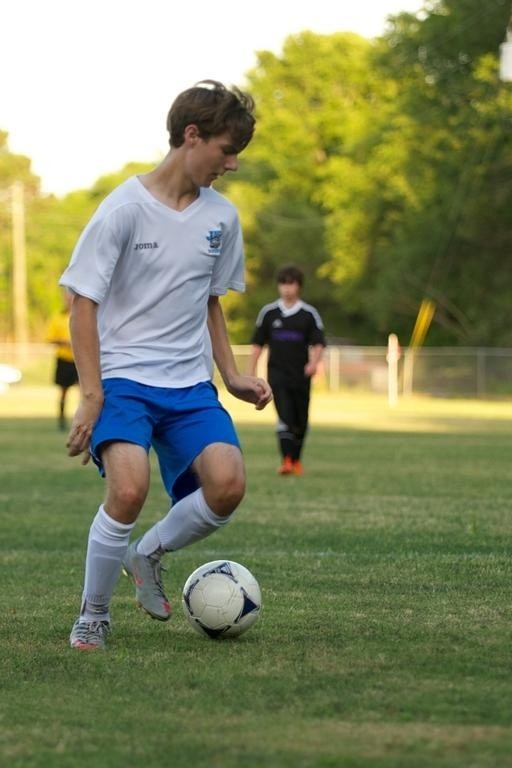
[120,535,173,625]
[277,456,306,475]
[68,615,114,652]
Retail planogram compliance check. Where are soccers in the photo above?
[179,559,261,640]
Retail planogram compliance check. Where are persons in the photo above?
[249,262,327,479]
[44,301,82,433]
[54,74,277,651]
